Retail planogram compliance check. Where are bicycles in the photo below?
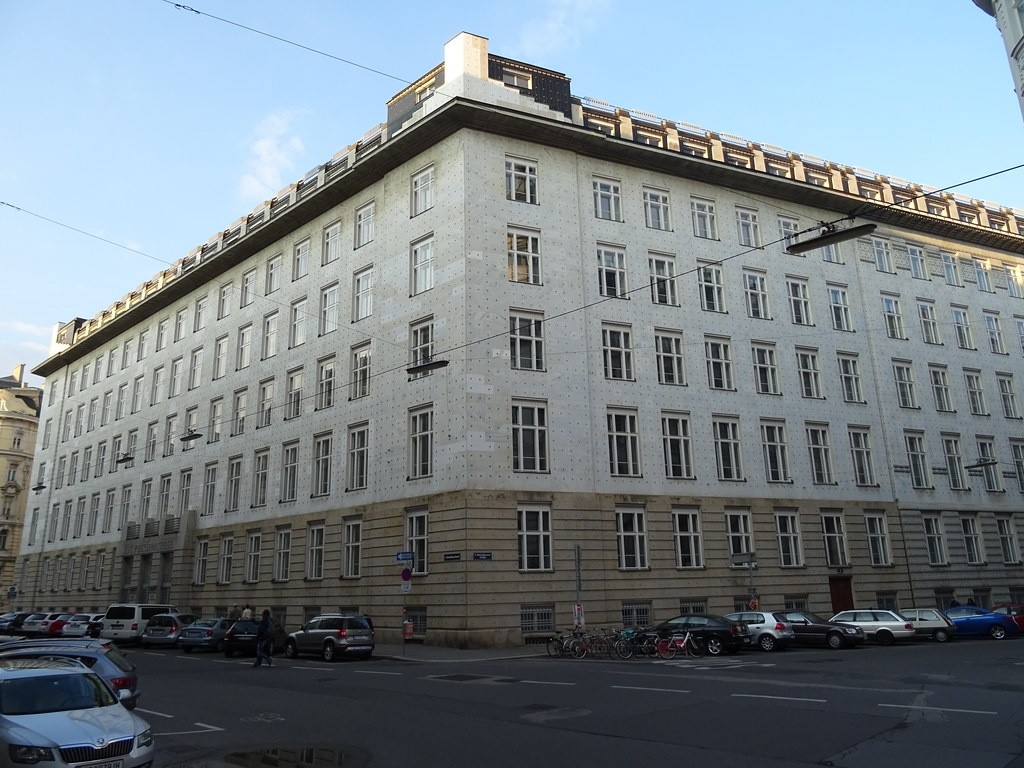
[546,624,706,659]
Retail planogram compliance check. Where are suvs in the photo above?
[0,655,155,768]
[0,640,141,711]
[287,613,376,662]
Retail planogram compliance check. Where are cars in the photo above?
[780,610,868,649]
[0,612,32,635]
[990,605,1024,632]
[0,636,124,657]
[86,617,105,638]
[943,605,1014,640]
[723,612,795,652]
[636,614,752,657]
[141,613,199,649]
[49,615,73,634]
[62,613,106,635]
[178,618,238,653]
[21,612,64,639]
[829,609,916,646]
[899,608,957,642]
[223,617,287,659]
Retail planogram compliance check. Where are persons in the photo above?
[950,596,960,608]
[967,598,977,606]
[229,603,252,619]
[749,593,759,610]
[251,609,272,668]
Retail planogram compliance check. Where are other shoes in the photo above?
[253,662,261,667]
[270,664,275,672]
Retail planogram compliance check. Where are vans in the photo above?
[100,603,182,645]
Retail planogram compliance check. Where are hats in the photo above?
[233,603,237,607]
